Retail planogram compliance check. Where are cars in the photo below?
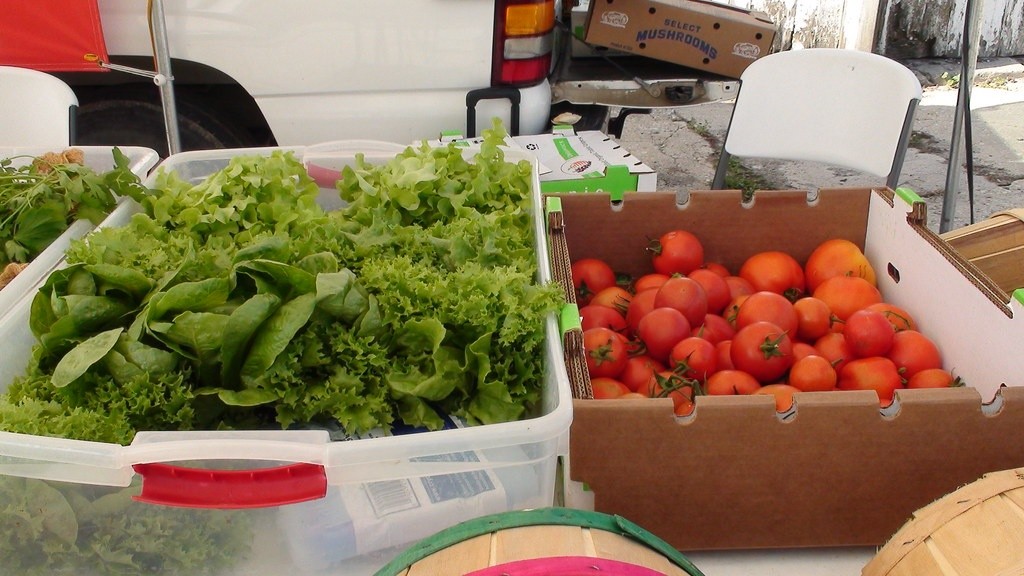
[0,1,739,156]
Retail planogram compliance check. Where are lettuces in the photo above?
[0,213,561,575]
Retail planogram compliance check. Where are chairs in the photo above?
[0,67,78,147]
[713,49,924,191]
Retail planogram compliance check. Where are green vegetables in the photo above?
[0,114,534,264]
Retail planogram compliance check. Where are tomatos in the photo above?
[569,229,954,410]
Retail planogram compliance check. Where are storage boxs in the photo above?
[583,0,775,79]
[0,128,1024,576]
[572,7,638,57]
